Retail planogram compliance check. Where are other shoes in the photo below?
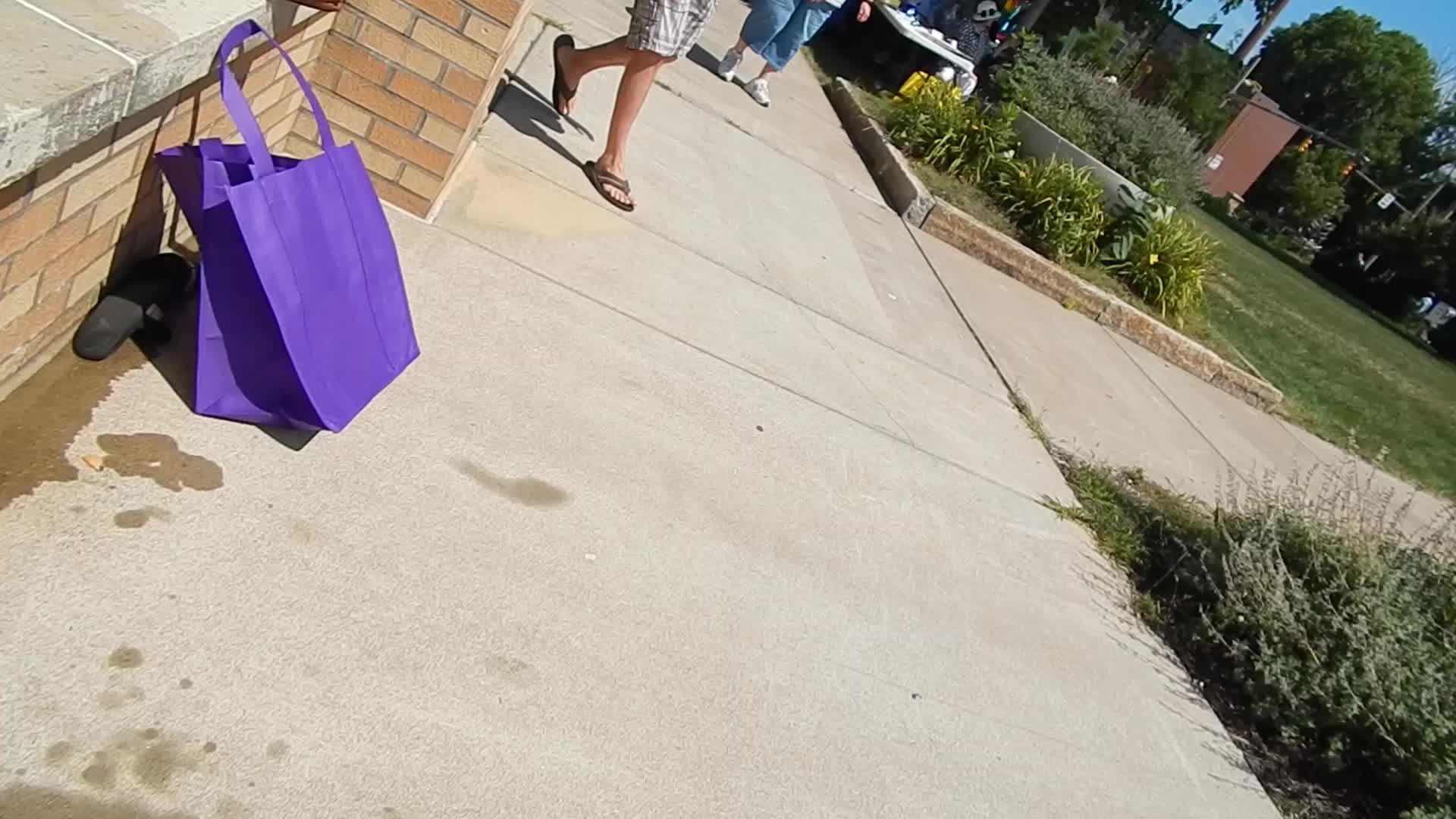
[70,253,196,360]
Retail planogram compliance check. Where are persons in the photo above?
[719,0,874,106]
[935,1,1001,95]
[1415,292,1435,313]
[552,0,719,210]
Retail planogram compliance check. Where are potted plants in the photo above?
[1001,45,1201,230]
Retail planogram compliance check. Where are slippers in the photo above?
[552,33,577,115]
[584,159,635,213]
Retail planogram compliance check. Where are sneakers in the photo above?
[717,49,743,81]
[746,78,770,107]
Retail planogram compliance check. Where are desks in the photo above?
[825,0,975,95]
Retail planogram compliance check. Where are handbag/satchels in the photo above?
[154,18,423,435]
